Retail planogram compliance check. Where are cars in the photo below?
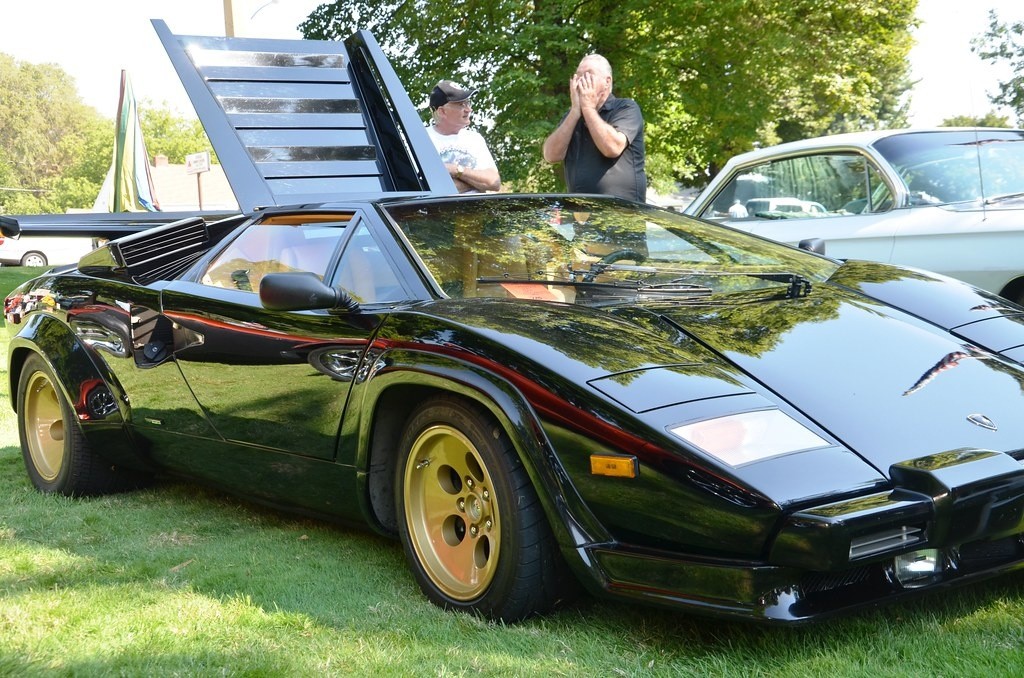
[681,126,1024,313]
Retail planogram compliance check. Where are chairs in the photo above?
[279,239,377,303]
[463,230,548,300]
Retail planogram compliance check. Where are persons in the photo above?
[408,78,501,195]
[543,55,649,258]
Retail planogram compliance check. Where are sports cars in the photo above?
[2,189,1024,631]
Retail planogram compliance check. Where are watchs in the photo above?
[456,166,464,179]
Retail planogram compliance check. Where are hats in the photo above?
[431,81,478,111]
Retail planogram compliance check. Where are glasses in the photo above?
[446,100,472,108]
